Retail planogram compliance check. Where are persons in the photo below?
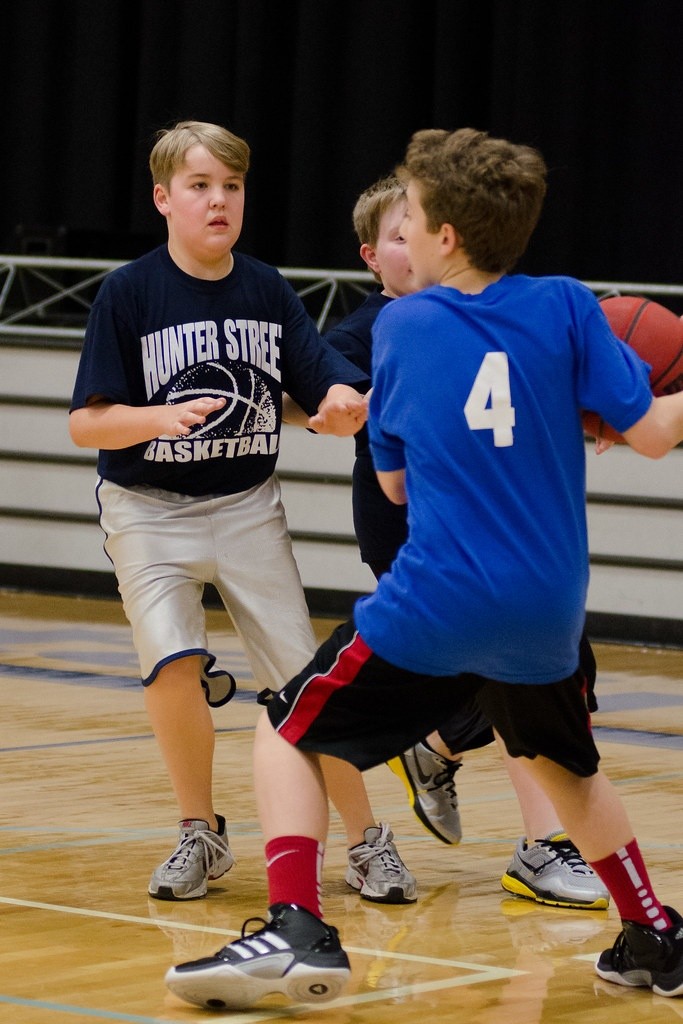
[165,129,683,1008]
[278,175,610,910]
[69,121,420,904]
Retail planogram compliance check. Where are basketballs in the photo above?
[577,295,683,445]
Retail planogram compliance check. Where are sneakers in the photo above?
[501,829,610,910]
[386,738,463,845]
[149,902,228,963]
[501,898,609,952]
[148,814,238,900]
[595,906,683,996]
[364,881,461,1002]
[345,820,419,903]
[593,978,683,1024]
[163,902,350,1010]
